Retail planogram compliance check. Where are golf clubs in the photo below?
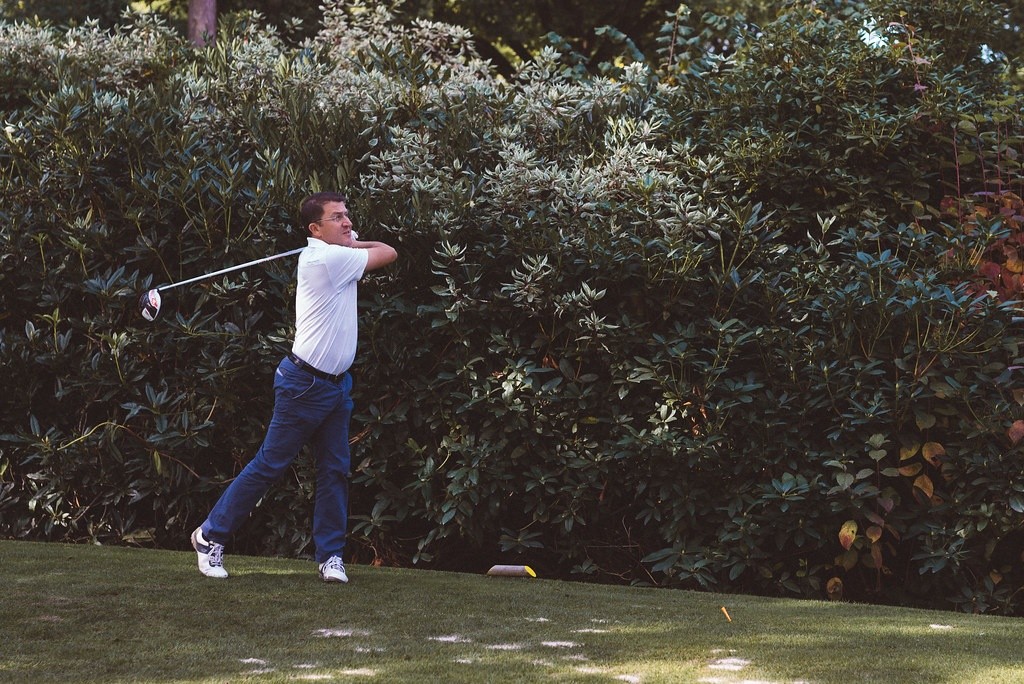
[137,230,360,323]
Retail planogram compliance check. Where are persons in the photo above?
[191,191,398,583]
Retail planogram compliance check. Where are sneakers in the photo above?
[191,527,229,579]
[318,556,349,583]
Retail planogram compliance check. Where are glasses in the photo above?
[319,212,349,223]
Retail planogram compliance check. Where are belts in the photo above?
[289,354,347,385]
